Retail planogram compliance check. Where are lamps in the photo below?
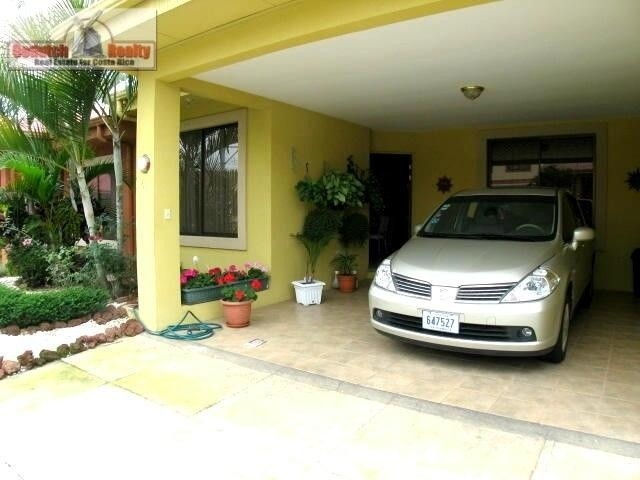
[461,86,485,101]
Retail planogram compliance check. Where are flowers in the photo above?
[180,260,269,288]
[221,278,263,302]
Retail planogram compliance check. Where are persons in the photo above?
[75,202,88,235]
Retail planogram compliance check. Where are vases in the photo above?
[221,299,254,328]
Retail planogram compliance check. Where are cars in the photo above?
[368,187,596,363]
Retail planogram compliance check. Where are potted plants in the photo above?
[329,251,360,294]
[289,230,340,307]
